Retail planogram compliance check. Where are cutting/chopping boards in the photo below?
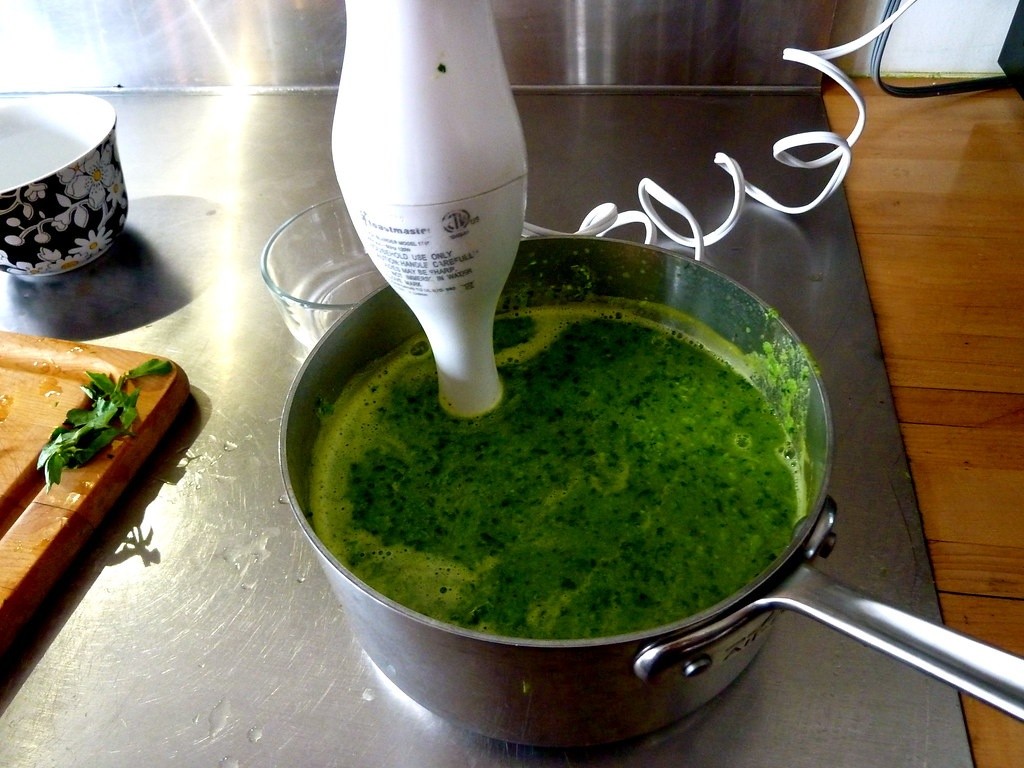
[0,330,191,651]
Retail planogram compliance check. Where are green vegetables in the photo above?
[36,358,174,494]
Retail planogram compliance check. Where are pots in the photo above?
[275,233,1024,749]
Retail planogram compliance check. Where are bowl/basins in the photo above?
[261,196,385,348]
[0,92,128,279]
